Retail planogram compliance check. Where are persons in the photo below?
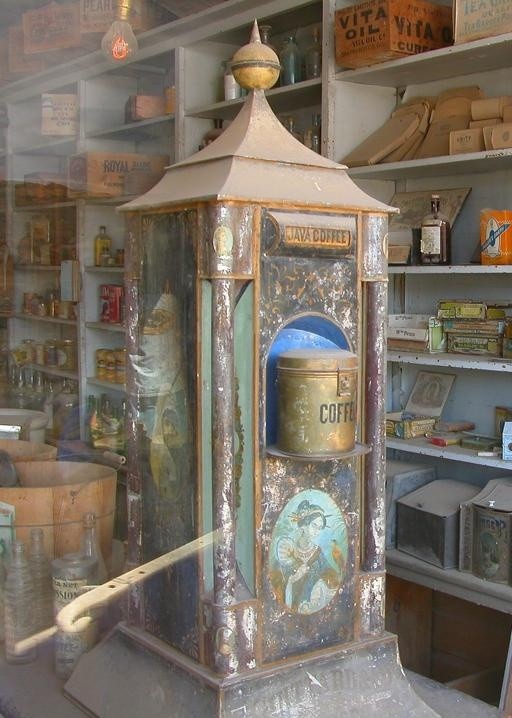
[273,498,342,615]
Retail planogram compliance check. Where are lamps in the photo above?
[9,26,48,76]
[21,0,101,68]
[100,0,139,67]
[79,0,154,37]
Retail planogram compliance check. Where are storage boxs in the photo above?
[395,478,480,570]
[479,210,512,266]
[14,151,171,206]
[450,0,512,46]
[128,94,165,120]
[39,91,77,137]
[386,457,439,551]
[386,313,448,354]
[334,1,451,68]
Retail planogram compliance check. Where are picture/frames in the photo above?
[388,186,472,230]
[404,369,457,418]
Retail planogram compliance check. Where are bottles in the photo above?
[418,193,452,266]
[0,512,111,680]
[7,364,78,441]
[280,107,323,159]
[94,219,124,268]
[221,17,321,101]
[85,387,130,456]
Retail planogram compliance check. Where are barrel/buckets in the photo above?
[275,347,358,455]
[0,409,119,586]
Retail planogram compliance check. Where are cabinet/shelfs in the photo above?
[0,0,512,712]
